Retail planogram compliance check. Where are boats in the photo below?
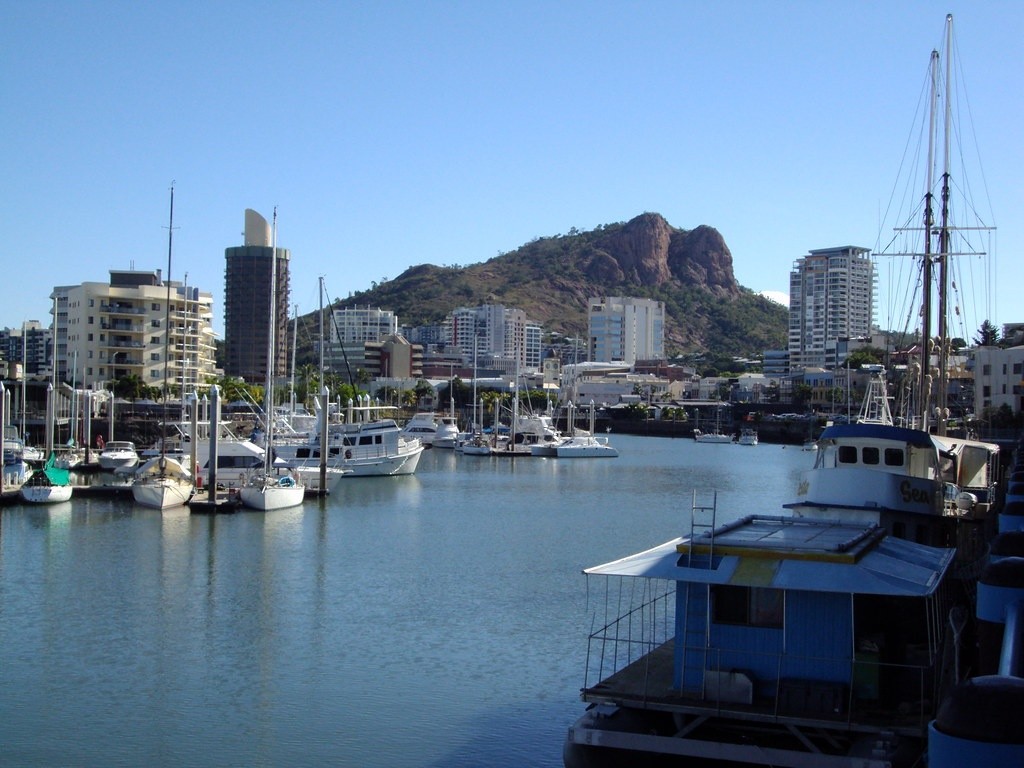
[398,410,621,458]
[98,440,140,470]
[274,416,426,477]
[142,432,344,495]
[777,171,1005,574]
[688,385,759,446]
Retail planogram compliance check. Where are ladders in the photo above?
[677,490,718,701]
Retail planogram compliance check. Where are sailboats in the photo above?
[239,201,307,512]
[12,296,75,506]
[128,190,194,512]
